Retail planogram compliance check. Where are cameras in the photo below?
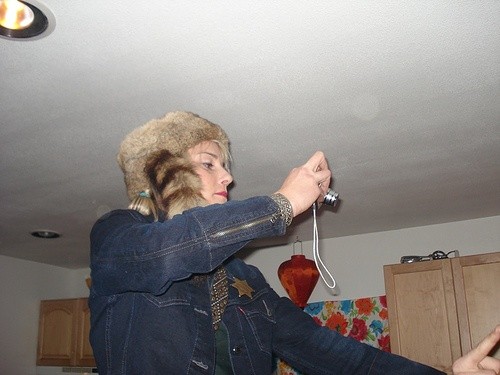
[310,187,339,214]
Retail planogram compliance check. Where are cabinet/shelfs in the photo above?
[383,253,500,375]
[36,297,96,367]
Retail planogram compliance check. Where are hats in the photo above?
[117,111,231,198]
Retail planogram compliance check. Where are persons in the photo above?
[88,111,500,375]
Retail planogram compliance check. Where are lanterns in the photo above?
[277,254,320,311]
